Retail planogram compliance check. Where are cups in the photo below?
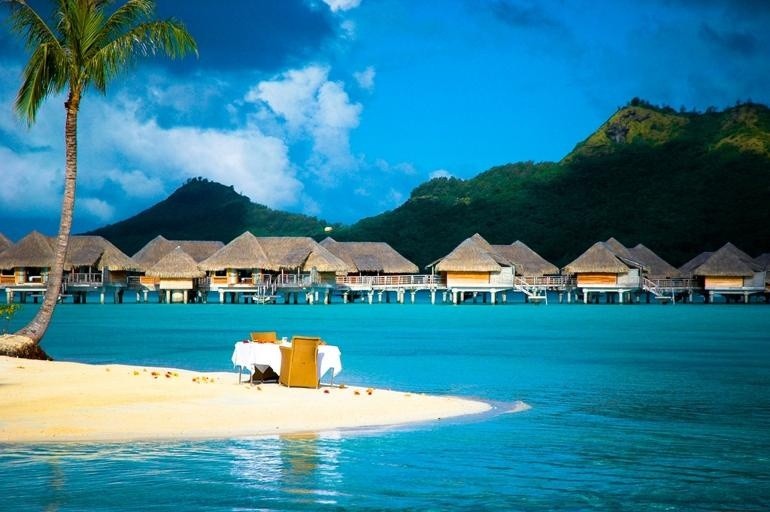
[282,337,288,343]
[243,336,249,343]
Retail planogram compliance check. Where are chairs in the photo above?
[249,331,320,390]
